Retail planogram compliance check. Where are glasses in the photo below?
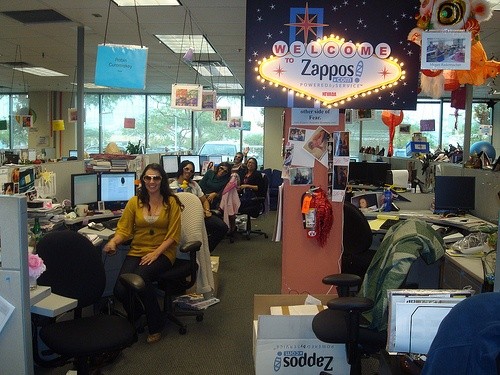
[219,166,228,174]
[143,174,162,184]
[183,167,195,174]
[235,156,242,159]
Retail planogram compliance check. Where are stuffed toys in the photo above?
[407,0,500,100]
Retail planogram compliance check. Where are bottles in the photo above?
[360,146,379,154]
[32,218,41,234]
[384,188,392,212]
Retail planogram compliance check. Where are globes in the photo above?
[470,141,496,160]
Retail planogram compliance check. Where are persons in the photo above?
[104,165,181,344]
[169,160,228,252]
[359,198,368,208]
[230,146,262,233]
[199,162,231,202]
[309,129,329,150]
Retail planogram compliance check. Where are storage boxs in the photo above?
[252,294,351,375]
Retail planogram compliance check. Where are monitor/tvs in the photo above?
[69,150,77,157]
[160,154,234,178]
[434,175,475,218]
[350,162,390,187]
[71,172,136,211]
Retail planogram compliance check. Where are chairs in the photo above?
[311,218,446,375]
[237,173,268,241]
[341,203,376,277]
[29,230,137,375]
[153,192,204,334]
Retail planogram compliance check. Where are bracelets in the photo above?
[206,209,210,211]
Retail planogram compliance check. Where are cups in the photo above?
[75,205,88,217]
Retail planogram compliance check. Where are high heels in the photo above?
[133,314,146,333]
[146,324,167,344]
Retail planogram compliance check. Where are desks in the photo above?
[0,152,205,375]
[359,209,499,290]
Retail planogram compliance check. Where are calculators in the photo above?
[88,222,106,231]
